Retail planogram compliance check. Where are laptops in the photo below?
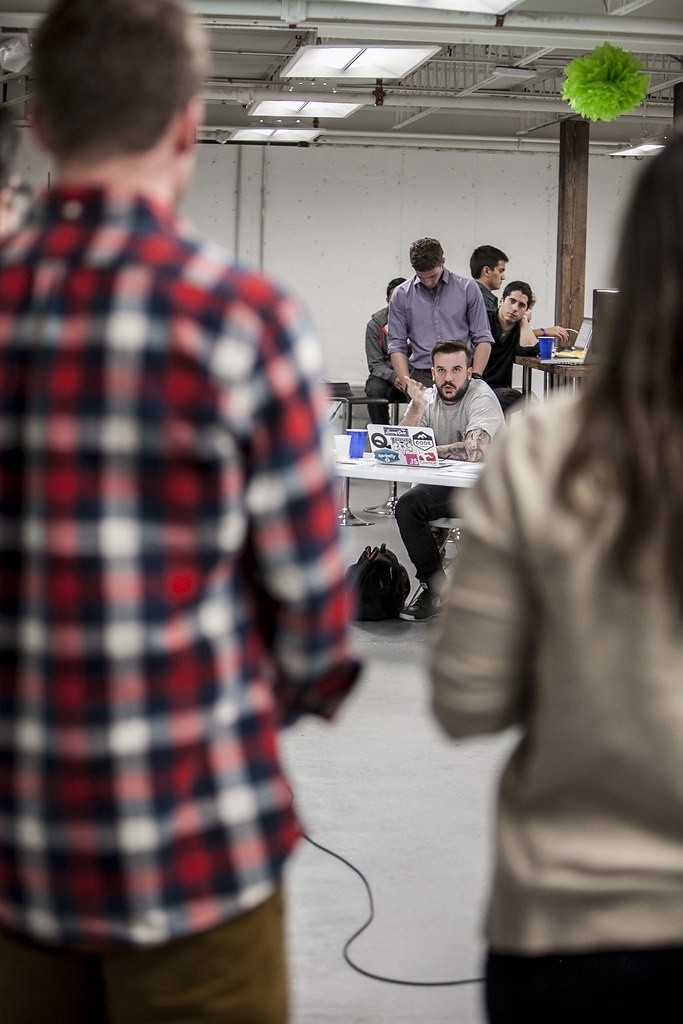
[366,424,458,469]
[564,316,593,353]
[542,289,620,365]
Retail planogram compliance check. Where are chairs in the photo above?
[406,518,473,612]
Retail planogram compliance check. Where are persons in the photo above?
[429,129,683,1024]
[1,0,367,1024]
[395,341,506,622]
[365,278,411,425]
[387,237,496,555]
[472,280,540,415]
[470,246,569,342]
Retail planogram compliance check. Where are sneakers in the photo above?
[405,576,451,620]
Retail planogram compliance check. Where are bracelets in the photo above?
[541,328,546,336]
[471,373,482,379]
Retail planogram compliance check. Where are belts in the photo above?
[414,368,431,373]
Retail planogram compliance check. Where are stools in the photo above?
[317,382,406,528]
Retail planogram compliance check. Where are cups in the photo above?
[538,337,555,359]
[334,435,352,458]
[346,429,369,458]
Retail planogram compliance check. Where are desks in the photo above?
[320,436,482,487]
[509,350,597,415]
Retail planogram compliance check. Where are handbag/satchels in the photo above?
[347,543,409,618]
